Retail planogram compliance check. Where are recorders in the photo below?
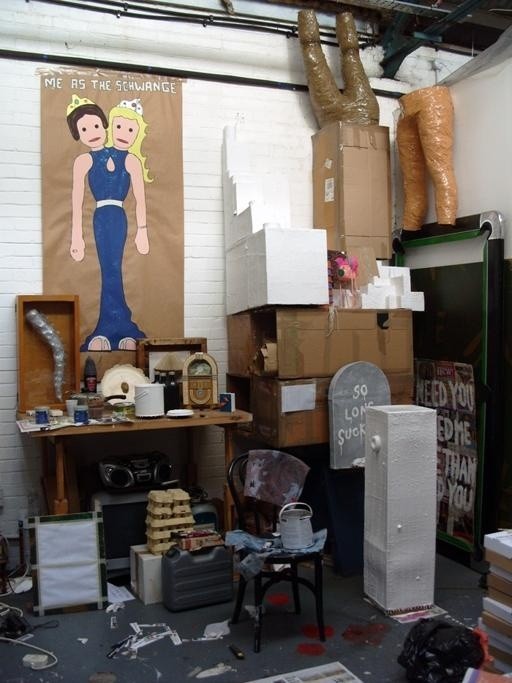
[93,449,174,491]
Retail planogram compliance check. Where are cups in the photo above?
[65,399,78,417]
[34,406,50,425]
[73,405,89,425]
[48,409,64,425]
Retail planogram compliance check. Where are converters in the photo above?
[23,654,49,668]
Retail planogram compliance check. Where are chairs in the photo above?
[227,450,327,653]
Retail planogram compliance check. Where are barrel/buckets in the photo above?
[135,385,165,418]
[276,502,316,551]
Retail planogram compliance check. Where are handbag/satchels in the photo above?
[396,617,484,683]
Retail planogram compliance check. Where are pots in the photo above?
[69,387,125,419]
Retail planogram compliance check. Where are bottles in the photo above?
[83,355,97,393]
[151,369,179,414]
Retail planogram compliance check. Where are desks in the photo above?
[14,406,252,554]
